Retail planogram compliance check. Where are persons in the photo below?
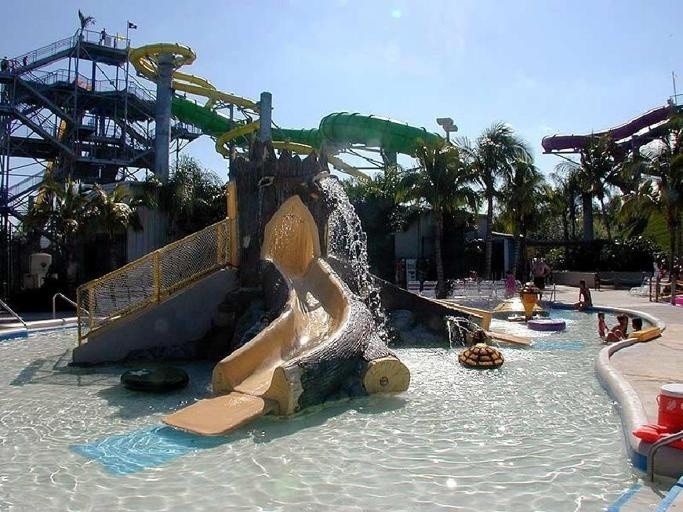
[99,28,107,44]
[531,253,550,288]
[606,314,629,342]
[598,312,609,338]
[505,272,516,299]
[22,55,29,66]
[573,280,592,312]
[593,273,601,291]
[631,317,642,331]
[0,57,8,72]
[2,85,9,104]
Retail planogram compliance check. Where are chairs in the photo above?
[407,280,522,301]
[629,276,669,298]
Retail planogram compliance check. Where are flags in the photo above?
[128,23,137,30]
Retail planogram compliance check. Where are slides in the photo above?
[330,255,533,347]
[161,253,372,436]
[542,106,681,175]
[128,42,446,182]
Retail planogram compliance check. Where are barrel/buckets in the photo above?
[655,383,683,449]
